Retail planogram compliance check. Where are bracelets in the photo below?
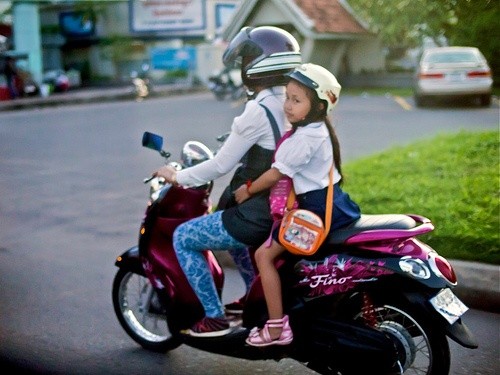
[246,179,252,196]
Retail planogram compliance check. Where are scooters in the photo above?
[110,131,480,375]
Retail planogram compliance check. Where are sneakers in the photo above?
[188,318,232,337]
[224,300,243,314]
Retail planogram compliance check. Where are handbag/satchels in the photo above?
[270,131,298,220]
[279,208,324,257]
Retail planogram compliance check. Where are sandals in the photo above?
[245,315,293,347]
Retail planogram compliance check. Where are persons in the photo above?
[153,26,303,337]
[232,64,361,347]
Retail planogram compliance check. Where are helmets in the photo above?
[283,63,342,116]
[222,26,301,85]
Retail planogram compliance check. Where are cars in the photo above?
[411,45,494,109]
